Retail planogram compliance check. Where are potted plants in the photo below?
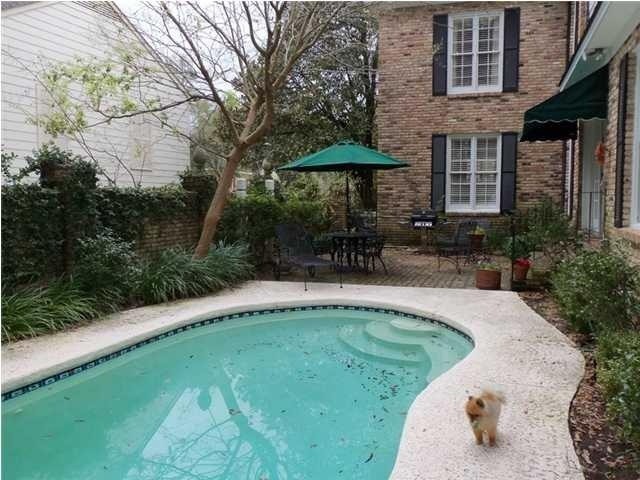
[466,225,532,290]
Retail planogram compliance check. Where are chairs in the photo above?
[272,215,392,292]
[434,218,478,275]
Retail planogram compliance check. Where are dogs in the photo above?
[465,388,510,448]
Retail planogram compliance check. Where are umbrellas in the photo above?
[276,139,410,266]
